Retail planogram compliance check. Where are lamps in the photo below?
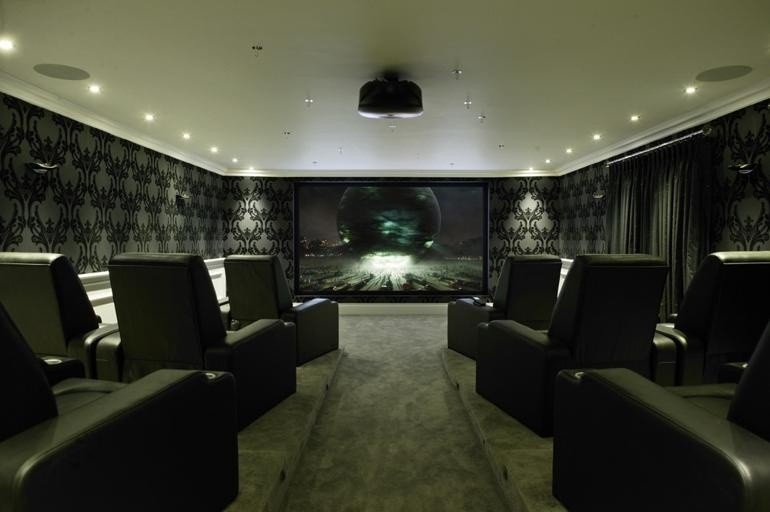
[29,161,58,174]
[357,76,423,119]
[727,159,757,174]
[176,192,192,199]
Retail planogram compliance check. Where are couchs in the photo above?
[552,320,770,512]
[477,252,669,437]
[0,251,119,380]
[218,254,339,367]
[0,302,239,512]
[448,254,562,361]
[656,251,769,385]
[96,252,296,432]
